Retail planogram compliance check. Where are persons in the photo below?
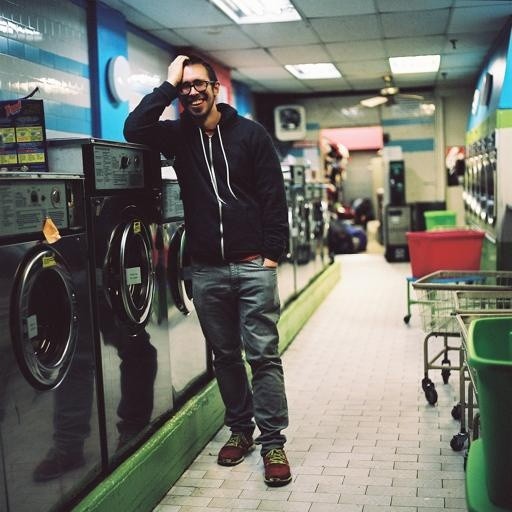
[29,196,162,484]
[121,53,293,487]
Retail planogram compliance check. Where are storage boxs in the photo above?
[404,232,484,280]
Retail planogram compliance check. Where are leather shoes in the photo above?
[216,430,256,466]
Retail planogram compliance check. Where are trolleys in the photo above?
[404,270,512,471]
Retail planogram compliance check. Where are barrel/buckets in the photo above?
[423,209,456,231]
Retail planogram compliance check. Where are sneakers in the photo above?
[263,446,292,488]
[33,440,85,480]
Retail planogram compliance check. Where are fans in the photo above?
[358,76,424,108]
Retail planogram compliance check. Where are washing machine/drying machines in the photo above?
[0,134,333,511]
[465,14,512,244]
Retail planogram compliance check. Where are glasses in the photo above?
[177,80,215,96]
